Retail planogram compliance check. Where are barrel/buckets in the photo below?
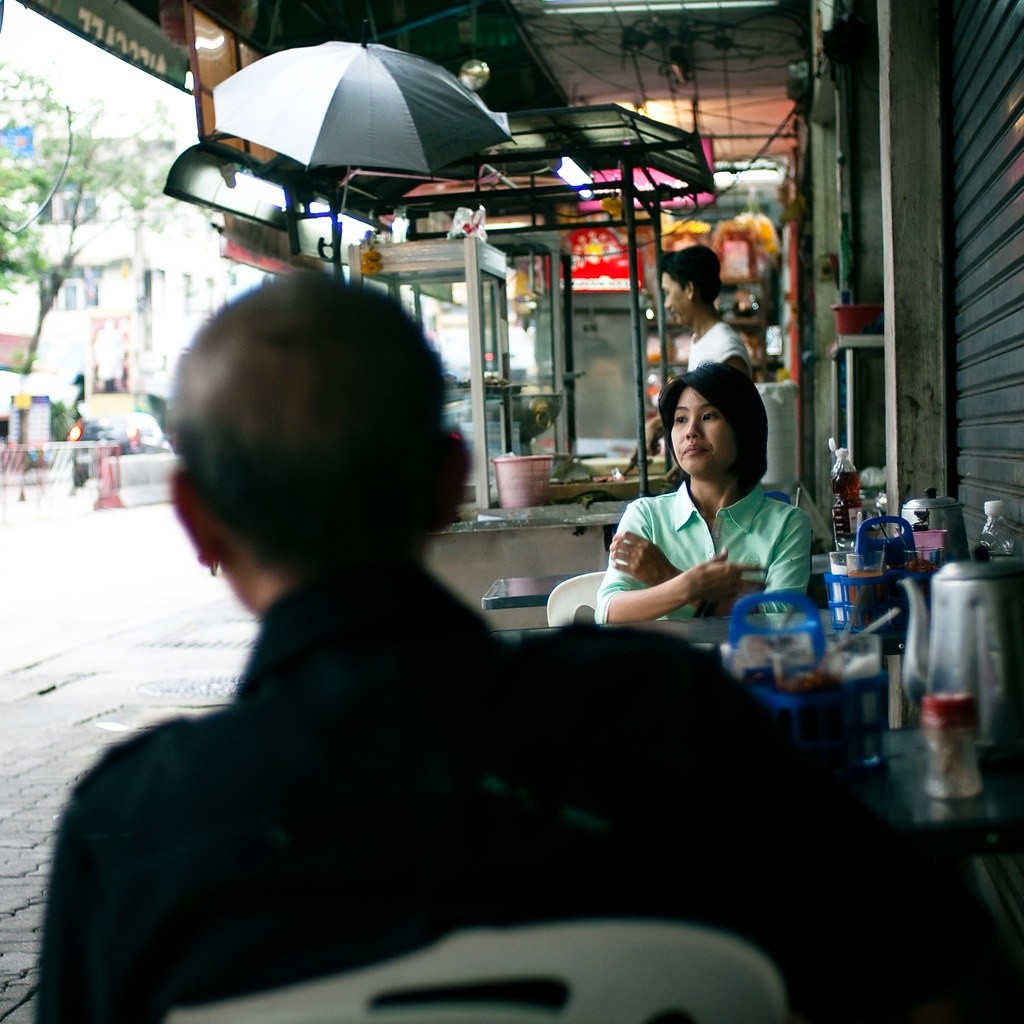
[492,454,551,508]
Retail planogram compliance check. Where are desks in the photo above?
[483,576,1016,823]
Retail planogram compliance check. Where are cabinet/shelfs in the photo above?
[489,233,576,477]
[350,239,515,516]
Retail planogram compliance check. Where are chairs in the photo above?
[545,573,606,626]
[163,924,786,1024]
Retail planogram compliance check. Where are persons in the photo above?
[595,361,811,625]
[629,244,751,490]
[37,270,995,1024]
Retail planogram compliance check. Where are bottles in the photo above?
[917,691,983,800]
[829,438,863,551]
[981,499,1015,559]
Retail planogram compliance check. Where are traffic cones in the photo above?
[92,460,125,508]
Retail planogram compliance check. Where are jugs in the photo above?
[898,545,1024,778]
[901,487,970,563]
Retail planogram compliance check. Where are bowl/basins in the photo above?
[755,379,798,492]
[833,304,882,335]
[458,419,523,503]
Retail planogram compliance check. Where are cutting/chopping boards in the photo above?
[578,455,673,475]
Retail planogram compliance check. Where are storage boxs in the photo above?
[913,529,948,549]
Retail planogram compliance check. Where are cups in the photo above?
[902,548,942,572]
[829,550,860,624]
[825,633,886,802]
[846,551,884,627]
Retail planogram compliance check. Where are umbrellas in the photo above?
[210,20,518,263]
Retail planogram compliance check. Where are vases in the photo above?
[832,303,876,336]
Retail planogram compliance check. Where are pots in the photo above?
[510,393,564,439]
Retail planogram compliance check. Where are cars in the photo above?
[64,414,173,487]
